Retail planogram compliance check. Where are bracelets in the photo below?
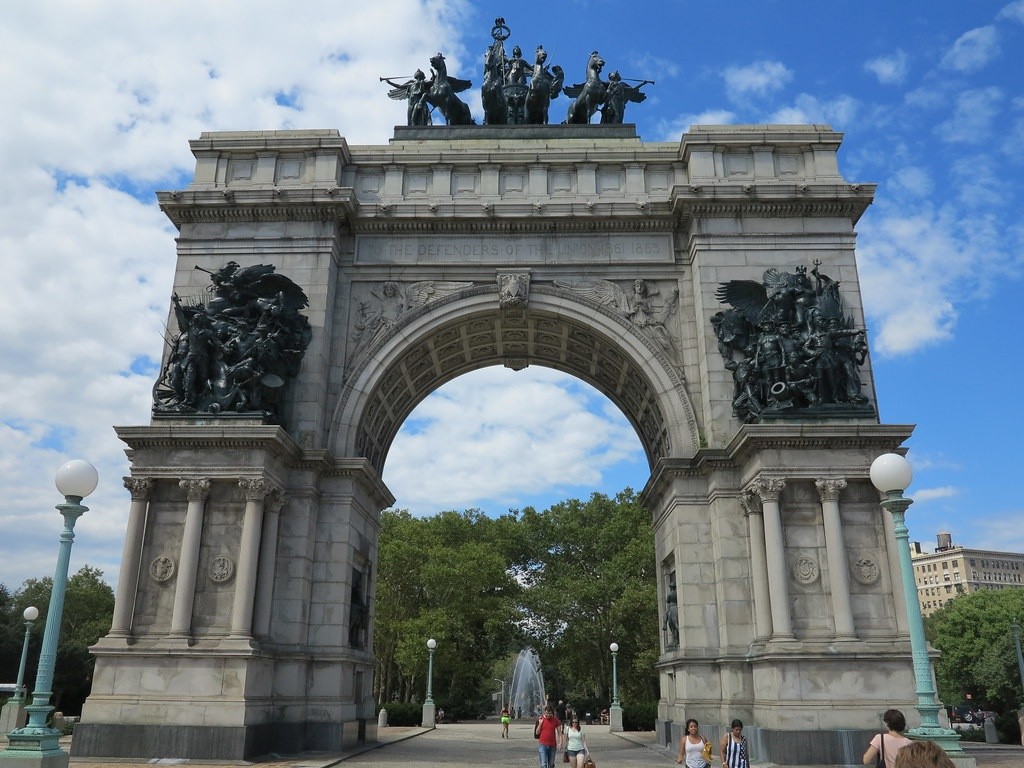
[722,762,726,767]
[676,760,682,764]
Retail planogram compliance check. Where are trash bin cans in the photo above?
[982,715,1002,745]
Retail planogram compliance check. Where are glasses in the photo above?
[572,722,577,723]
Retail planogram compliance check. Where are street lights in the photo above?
[495,679,504,709]
[869,453,966,754]
[421,639,437,728]
[0,606,40,743]
[0,460,99,752]
[610,643,623,732]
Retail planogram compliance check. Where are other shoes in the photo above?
[501,732,504,738]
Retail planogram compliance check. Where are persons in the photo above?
[499,703,523,738]
[863,709,957,768]
[498,46,551,85]
[379,281,410,325]
[600,71,648,123]
[720,719,750,768]
[626,279,657,327]
[600,709,609,725]
[535,700,590,768]
[435,707,444,724]
[675,719,711,768]
[753,276,870,405]
[661,583,677,632]
[386,69,435,126]
[167,274,279,412]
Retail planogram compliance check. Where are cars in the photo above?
[953,702,980,723]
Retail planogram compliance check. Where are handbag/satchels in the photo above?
[563,751,569,763]
[584,756,596,768]
[876,734,886,768]
[534,715,543,739]
